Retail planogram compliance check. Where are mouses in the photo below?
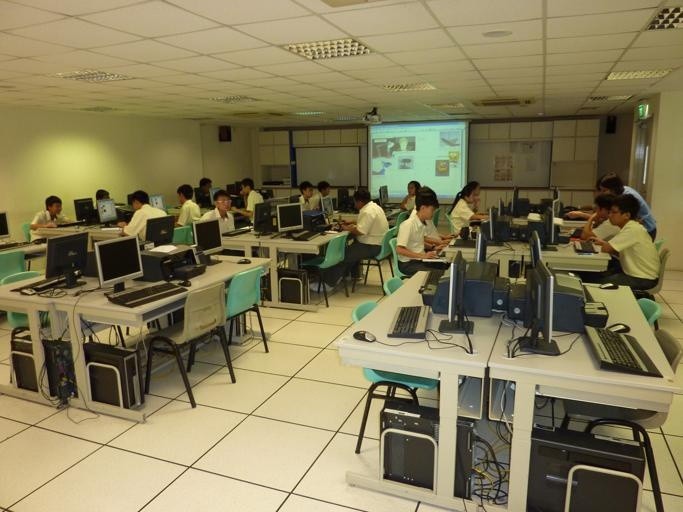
[607,323,630,333]
[600,282,618,289]
[21,288,36,295]
[35,240,41,244]
[237,259,250,264]
[20,288,36,295]
[353,331,376,342]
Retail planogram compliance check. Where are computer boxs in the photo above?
[10,336,143,409]
[380,398,645,512]
[279,270,308,304]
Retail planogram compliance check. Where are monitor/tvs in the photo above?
[253,202,273,236]
[337,187,349,210]
[358,186,369,192]
[148,194,167,211]
[226,181,243,194]
[192,219,223,266]
[0,212,10,238]
[276,203,305,239]
[289,195,301,203]
[209,187,224,206]
[94,236,143,297]
[437,184,561,356]
[379,186,389,208]
[46,232,88,289]
[127,194,133,206]
[320,195,335,218]
[145,216,175,246]
[73,198,116,228]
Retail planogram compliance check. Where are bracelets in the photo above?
[431,244,434,250]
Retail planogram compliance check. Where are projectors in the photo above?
[361,112,382,124]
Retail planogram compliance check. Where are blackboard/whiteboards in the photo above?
[296,146,361,188]
[468,140,552,189]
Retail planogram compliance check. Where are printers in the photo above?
[422,262,509,317]
[141,243,206,280]
[508,275,608,334]
[85,251,102,277]
[256,188,274,199]
[302,210,333,234]
[231,197,245,208]
[115,206,135,223]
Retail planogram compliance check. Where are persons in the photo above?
[398,180,422,211]
[117,189,168,242]
[229,178,264,225]
[298,181,313,211]
[28,195,73,243]
[447,180,490,232]
[563,176,603,220]
[396,193,446,278]
[200,190,259,258]
[579,194,622,241]
[586,193,660,301]
[595,171,657,244]
[172,183,200,227]
[308,189,388,292]
[197,179,214,206]
[309,181,329,212]
[409,187,462,247]
[95,189,109,201]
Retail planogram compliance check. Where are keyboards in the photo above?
[57,222,80,227]
[10,269,81,292]
[387,305,430,339]
[222,227,250,237]
[585,325,663,376]
[109,283,189,308]
[425,267,444,285]
[584,285,594,303]
[0,241,30,250]
[574,240,594,252]
[295,232,320,241]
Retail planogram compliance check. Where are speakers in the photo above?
[219,125,231,142]
[607,116,616,134]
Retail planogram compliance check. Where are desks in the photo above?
[337,207,682,512]
[0,199,399,425]
